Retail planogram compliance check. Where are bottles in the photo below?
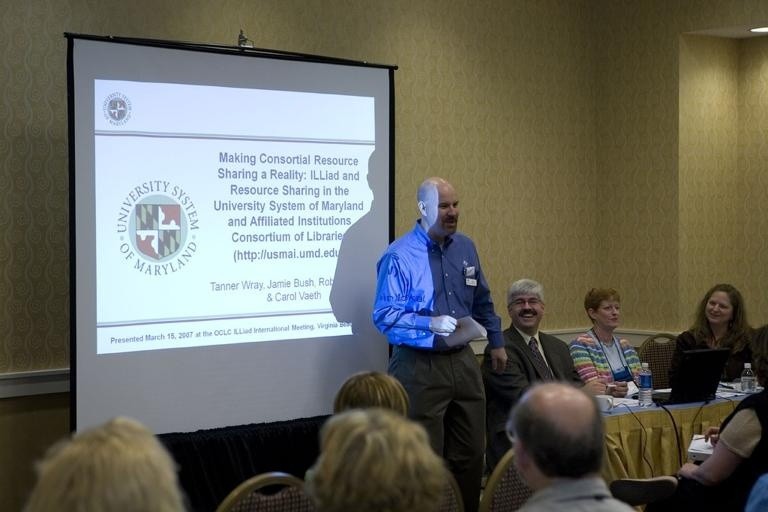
[740,363,755,394]
[638,363,652,408]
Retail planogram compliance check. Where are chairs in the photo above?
[219,471,322,512]
[475,447,534,512]
[442,470,466,512]
[638,331,683,391]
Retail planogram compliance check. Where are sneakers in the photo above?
[610,477,678,506]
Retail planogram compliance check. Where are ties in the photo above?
[528,336,554,383]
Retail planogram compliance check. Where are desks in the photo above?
[587,375,761,475]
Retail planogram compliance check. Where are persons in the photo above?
[568,289,646,397]
[610,376,768,512]
[22,417,184,512]
[333,371,410,417]
[483,277,627,462]
[513,381,640,510]
[672,285,760,402]
[372,177,508,512]
[309,409,460,512]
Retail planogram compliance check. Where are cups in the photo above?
[596,395,613,412]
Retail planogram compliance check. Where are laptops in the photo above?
[631,348,731,405]
